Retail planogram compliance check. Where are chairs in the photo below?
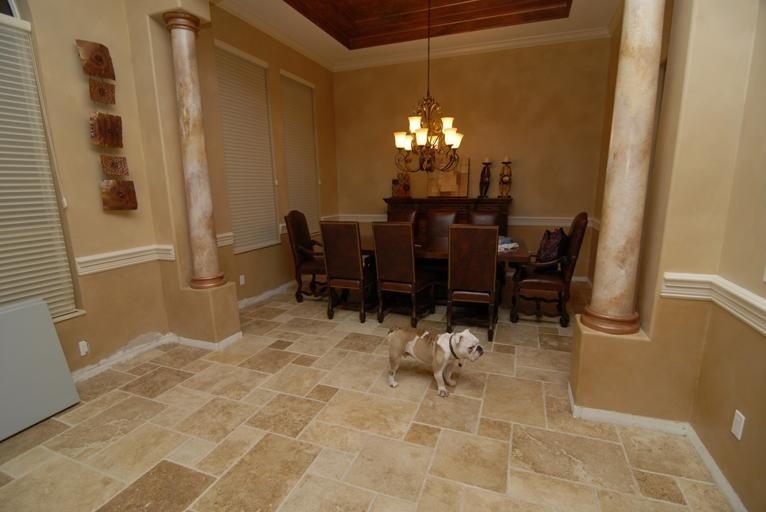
[317,219,373,323]
[508,212,588,328]
[369,222,438,328]
[284,210,340,305]
[446,223,499,340]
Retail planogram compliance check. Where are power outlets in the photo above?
[729,409,746,441]
[238,273,246,286]
[77,339,90,357]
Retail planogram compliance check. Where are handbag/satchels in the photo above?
[529,226,568,278]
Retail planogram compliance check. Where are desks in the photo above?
[327,234,531,302]
[382,196,514,240]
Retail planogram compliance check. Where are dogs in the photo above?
[386,326,485,397]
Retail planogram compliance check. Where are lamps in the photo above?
[393,0,465,174]
